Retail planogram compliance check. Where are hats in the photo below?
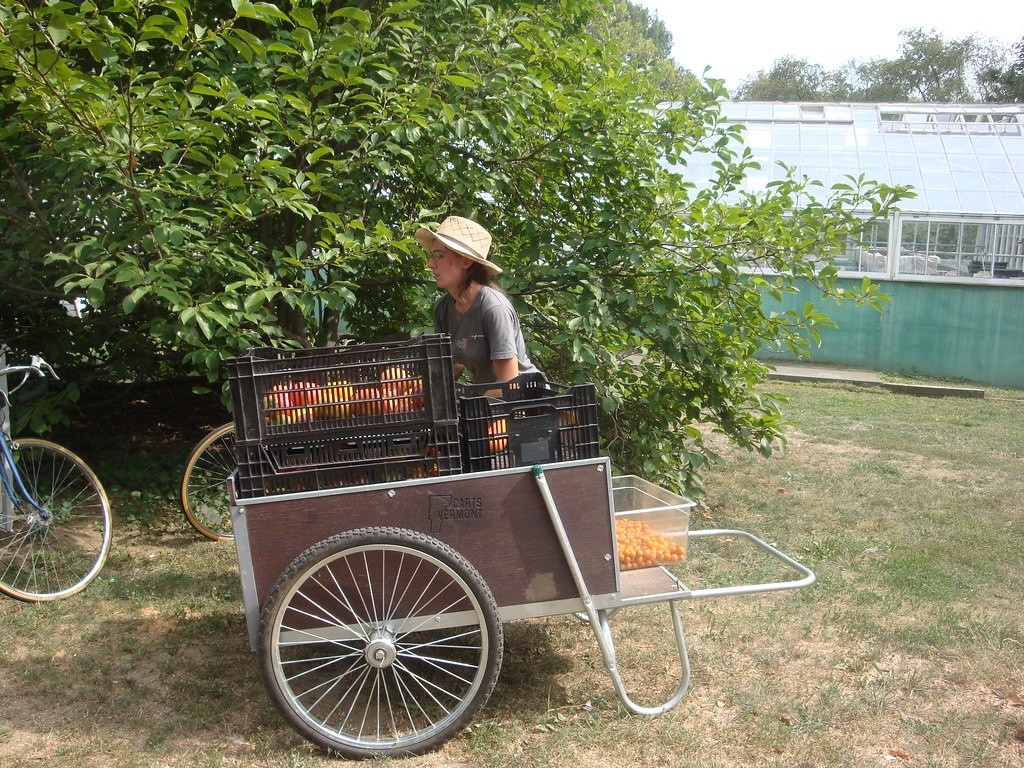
[415,215,504,276]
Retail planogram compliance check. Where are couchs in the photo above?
[856,246,936,275]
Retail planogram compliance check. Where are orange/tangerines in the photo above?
[614,517,686,571]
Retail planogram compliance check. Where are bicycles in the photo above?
[180,420,238,541]
[0,352,113,604]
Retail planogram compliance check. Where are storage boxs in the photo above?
[222,333,463,445]
[235,422,464,499]
[451,372,602,475]
[611,473,695,570]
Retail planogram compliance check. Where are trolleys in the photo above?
[227,456,816,760]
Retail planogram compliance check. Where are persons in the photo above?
[414,211,552,395]
